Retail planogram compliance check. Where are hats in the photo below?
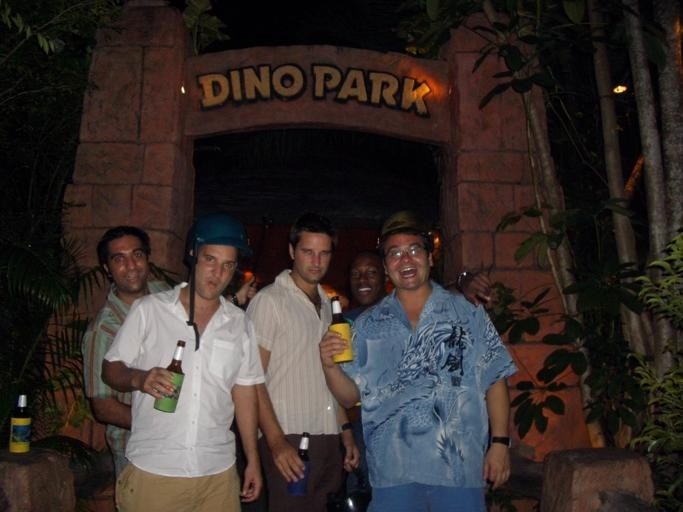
[187,213,253,258]
[377,210,431,238]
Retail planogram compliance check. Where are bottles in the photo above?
[8,392,33,454]
[326,295,353,365]
[286,431,314,498]
[153,339,185,414]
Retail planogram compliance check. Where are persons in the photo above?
[100,215,264,512]
[244,210,361,512]
[81,225,257,512]
[318,211,518,512]
[338,252,492,512]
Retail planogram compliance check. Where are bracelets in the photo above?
[456,270,473,290]
[490,436,512,449]
[229,293,239,307]
[340,423,353,432]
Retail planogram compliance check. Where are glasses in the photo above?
[390,246,419,260]
